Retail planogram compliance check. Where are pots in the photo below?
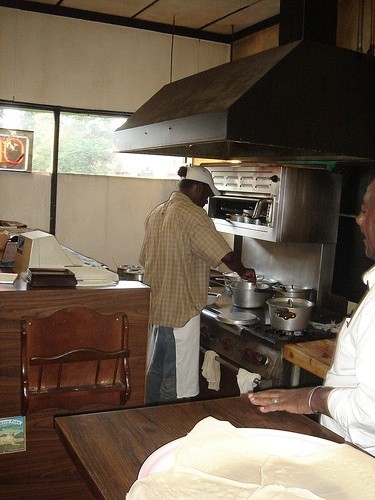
[221,205,267,225]
[214,270,315,332]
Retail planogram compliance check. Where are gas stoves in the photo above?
[200,286,343,381]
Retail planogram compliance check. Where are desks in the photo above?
[53,397,375,500]
[283,338,338,390]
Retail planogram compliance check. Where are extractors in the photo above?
[112,0,375,163]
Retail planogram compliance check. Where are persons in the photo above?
[139,164,256,406]
[247,181,375,458]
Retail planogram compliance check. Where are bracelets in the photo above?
[308,385,323,415]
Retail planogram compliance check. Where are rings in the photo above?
[271,397,279,404]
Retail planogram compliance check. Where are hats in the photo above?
[181,165,221,195]
